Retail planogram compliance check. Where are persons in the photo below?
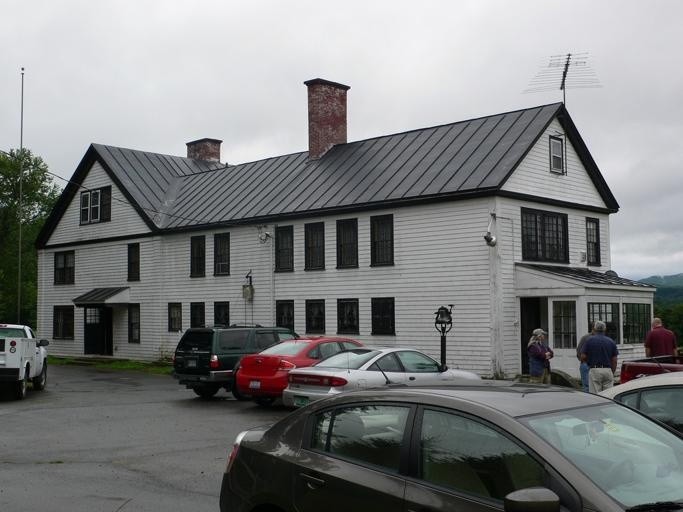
[574,321,598,390]
[525,328,554,385]
[644,316,679,360]
[580,320,618,393]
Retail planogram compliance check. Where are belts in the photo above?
[591,365,611,368]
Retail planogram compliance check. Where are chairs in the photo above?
[427,426,491,498]
[497,432,550,490]
[330,413,374,462]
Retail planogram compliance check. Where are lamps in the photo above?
[241,270,255,301]
[483,212,497,248]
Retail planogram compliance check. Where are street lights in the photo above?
[433,304,455,371]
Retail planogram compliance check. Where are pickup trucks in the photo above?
[619,356,683,386]
[0,322,49,401]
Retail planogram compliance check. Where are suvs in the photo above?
[171,324,302,399]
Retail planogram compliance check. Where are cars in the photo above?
[217,372,683,512]
[584,372,683,435]
[281,348,482,412]
[236,336,394,408]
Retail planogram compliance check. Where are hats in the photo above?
[532,328,549,336]
